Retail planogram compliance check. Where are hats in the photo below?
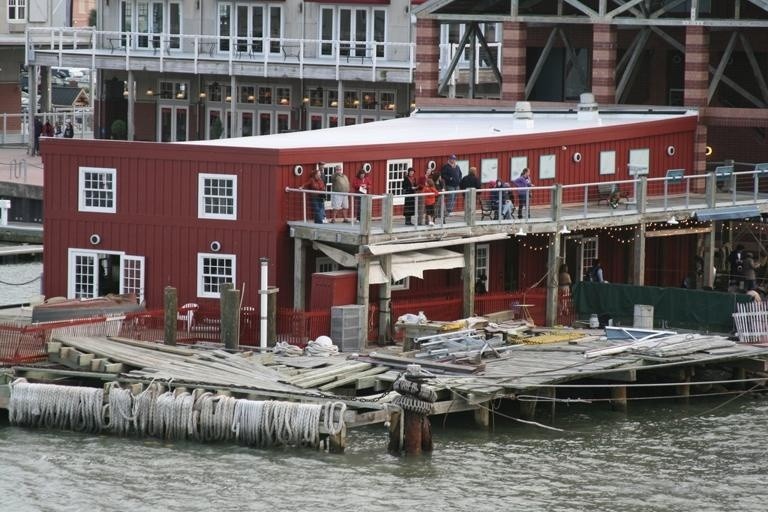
[449,154,457,160]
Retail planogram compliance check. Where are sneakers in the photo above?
[343,218,352,223]
[330,219,335,223]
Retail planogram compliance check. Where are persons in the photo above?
[726,243,744,287]
[42,118,55,137]
[352,168,372,224]
[27,116,44,155]
[330,164,351,224]
[474,274,489,317]
[590,257,608,284]
[297,170,329,224]
[558,263,572,316]
[741,251,759,289]
[401,152,535,225]
[62,122,74,138]
